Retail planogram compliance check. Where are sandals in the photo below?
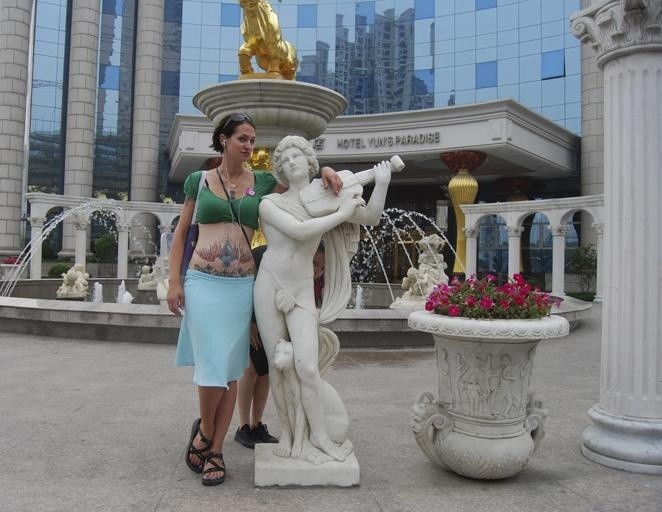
[184,418,225,486]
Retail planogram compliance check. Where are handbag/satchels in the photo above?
[181,170,206,275]
[249,312,268,376]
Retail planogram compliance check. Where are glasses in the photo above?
[222,113,251,130]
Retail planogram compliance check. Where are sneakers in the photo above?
[234,421,279,449]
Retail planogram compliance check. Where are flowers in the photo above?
[425,272,562,321]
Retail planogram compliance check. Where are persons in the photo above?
[167,114,342,487]
[252,135,395,462]
